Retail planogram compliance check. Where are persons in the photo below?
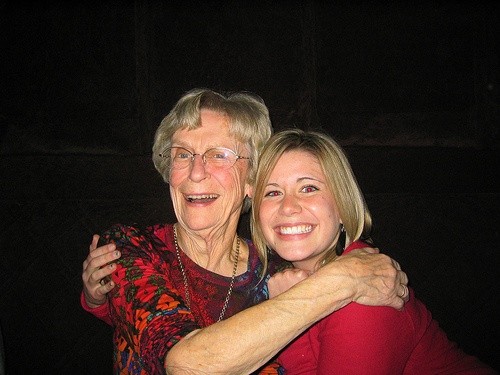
[94,88,410,374]
[80,124,500,375]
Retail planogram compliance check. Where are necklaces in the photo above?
[172,223,239,324]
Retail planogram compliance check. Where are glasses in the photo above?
[158,146,251,171]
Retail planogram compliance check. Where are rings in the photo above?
[398,285,405,297]
